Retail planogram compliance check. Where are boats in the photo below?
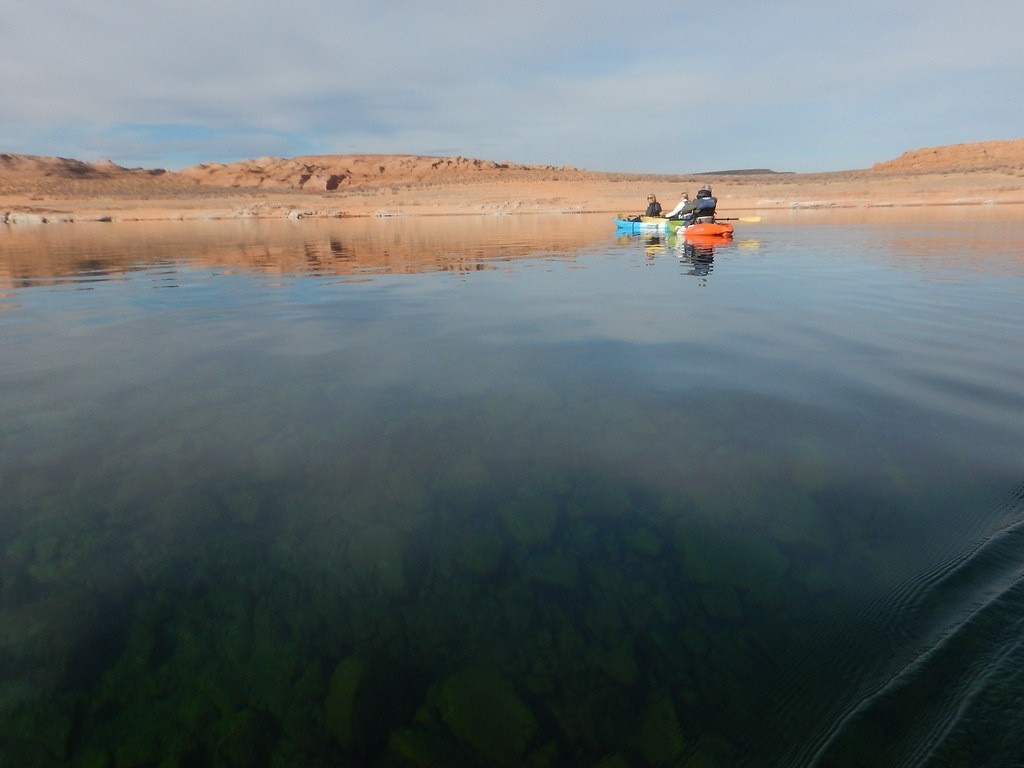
[615,214,734,238]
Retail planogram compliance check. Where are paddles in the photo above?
[618,214,639,218]
[654,214,667,218]
[668,215,762,222]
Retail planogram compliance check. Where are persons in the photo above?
[623,194,662,222]
[661,192,693,219]
[682,184,717,225]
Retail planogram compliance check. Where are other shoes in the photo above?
[628,218,631,222]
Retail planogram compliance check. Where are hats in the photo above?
[702,185,712,193]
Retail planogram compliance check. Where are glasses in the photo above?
[647,197,652,200]
